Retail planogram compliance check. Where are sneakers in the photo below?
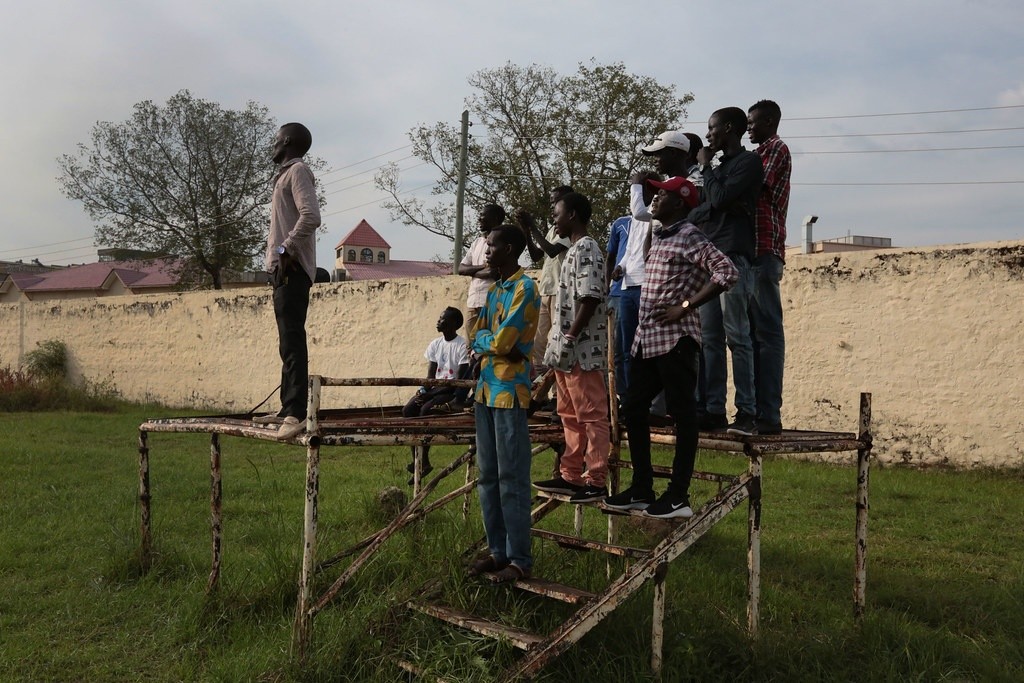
[643,491,694,519]
[726,416,759,435]
[569,486,608,503]
[531,478,581,496]
[603,486,655,510]
[696,414,729,433]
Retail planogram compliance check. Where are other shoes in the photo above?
[758,421,782,434]
[407,464,433,485]
[253,412,284,424]
[278,416,308,439]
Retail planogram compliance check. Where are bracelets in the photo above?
[566,334,576,341]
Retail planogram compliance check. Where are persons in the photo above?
[431,204,506,415]
[253,123,321,438]
[469,224,542,587]
[600,174,739,520]
[604,131,728,431]
[530,191,610,503]
[685,107,765,436]
[747,100,792,435]
[401,306,470,484]
[516,183,575,425]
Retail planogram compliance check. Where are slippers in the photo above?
[474,556,499,573]
[489,563,524,587]
[429,403,455,413]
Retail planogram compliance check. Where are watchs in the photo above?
[682,300,695,314]
[277,246,290,259]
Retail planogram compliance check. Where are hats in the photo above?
[642,130,691,155]
[646,176,699,210]
